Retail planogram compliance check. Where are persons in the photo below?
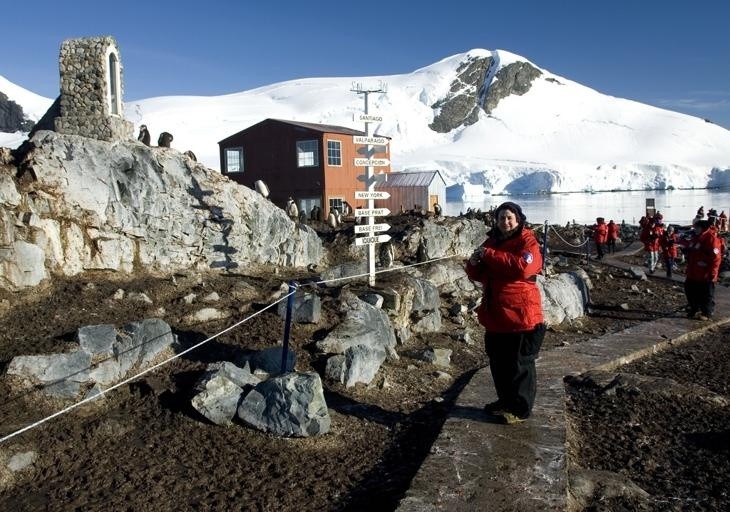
[465,202,546,425]
[639,211,677,278]
[591,218,619,260]
[681,220,722,321]
[697,206,727,231]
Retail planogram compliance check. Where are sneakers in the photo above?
[686,308,712,322]
[482,399,529,425]
